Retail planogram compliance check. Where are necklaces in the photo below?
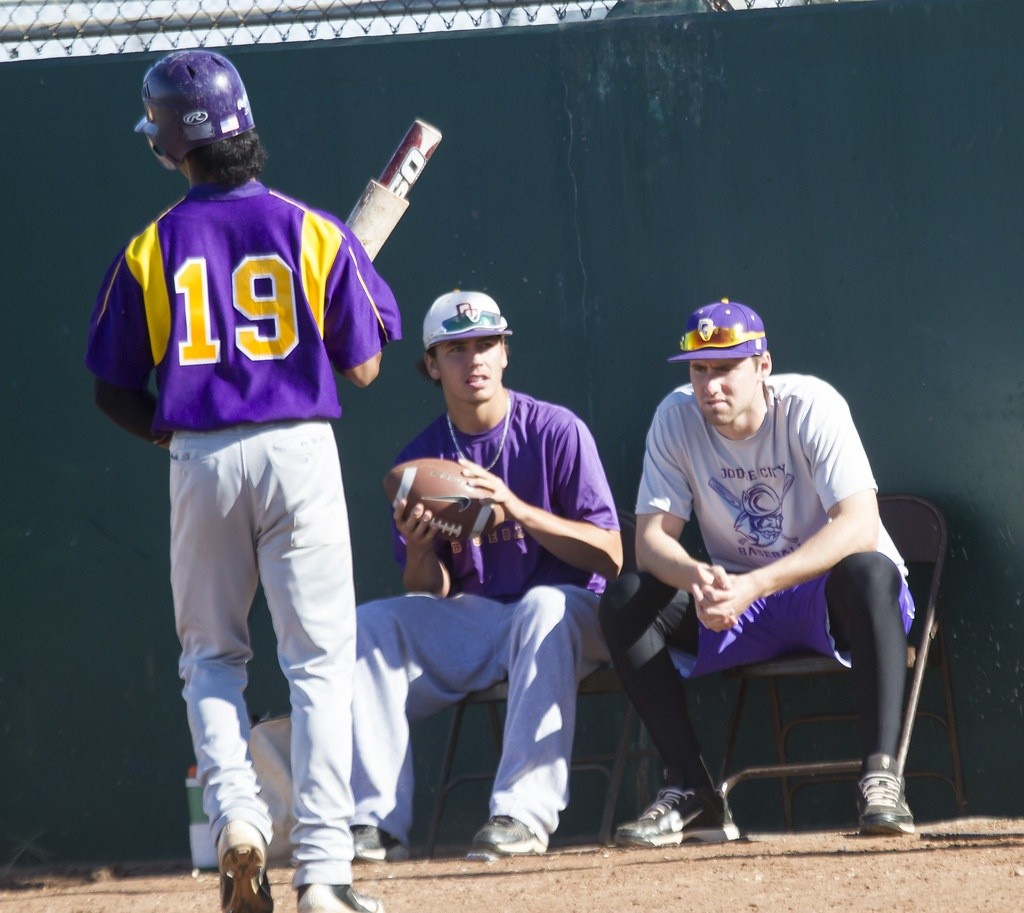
[446,392,511,472]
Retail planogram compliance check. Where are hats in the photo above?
[422,288,512,349]
[667,298,767,361]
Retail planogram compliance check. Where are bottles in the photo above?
[185,766,220,869]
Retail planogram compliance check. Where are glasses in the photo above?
[679,326,764,351]
[421,310,508,339]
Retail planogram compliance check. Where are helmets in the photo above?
[133,50,257,170]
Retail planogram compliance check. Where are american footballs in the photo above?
[382,456,507,542]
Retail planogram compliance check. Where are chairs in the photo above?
[711,496,970,829]
[426,510,650,859]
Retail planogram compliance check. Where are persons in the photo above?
[84,50,402,913]
[354,288,624,863]
[597,298,917,848]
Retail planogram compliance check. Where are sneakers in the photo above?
[350,824,410,859]
[614,780,740,848]
[856,753,915,834]
[217,821,274,913]
[465,815,546,858]
[296,883,385,913]
